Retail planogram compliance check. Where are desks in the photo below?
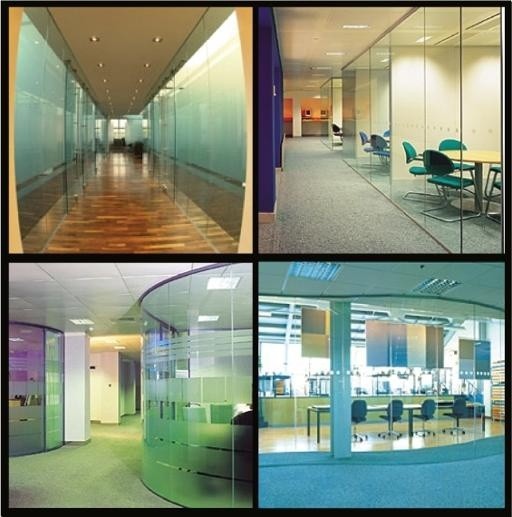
[419,150,501,215]
[306,401,485,443]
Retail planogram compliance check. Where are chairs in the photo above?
[332,124,343,140]
[350,398,467,442]
[360,130,390,176]
[402,139,483,223]
[485,166,501,224]
